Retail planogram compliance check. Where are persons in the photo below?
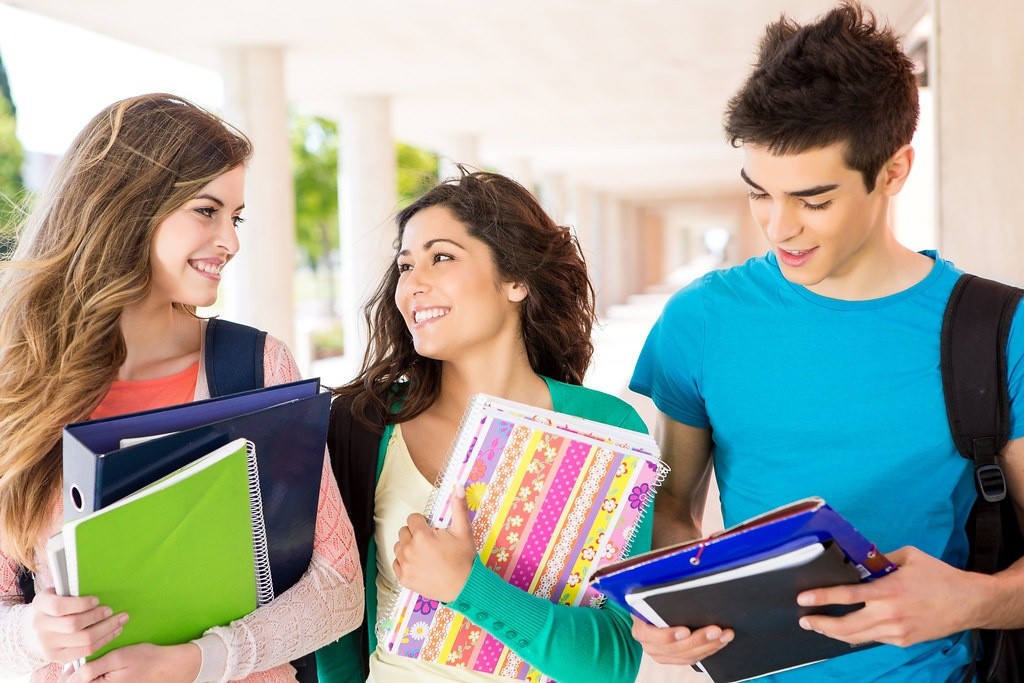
[0,94,366,683]
[316,166,653,683]
[631,0,1024,683]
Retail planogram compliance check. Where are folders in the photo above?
[62,376,332,598]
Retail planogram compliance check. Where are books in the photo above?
[59,438,288,672]
[383,393,898,682]
